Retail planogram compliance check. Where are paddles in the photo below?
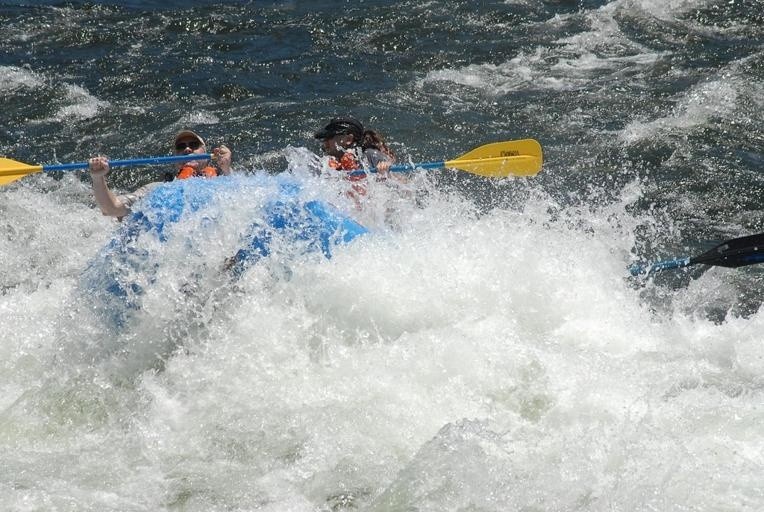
[340,140,542,177]
[631,233,764,275]
[0,154,213,187]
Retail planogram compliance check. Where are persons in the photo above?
[88,129,237,222]
[313,117,415,230]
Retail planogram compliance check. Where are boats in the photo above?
[89,169,377,337]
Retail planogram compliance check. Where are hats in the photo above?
[315,116,363,141]
[173,130,205,146]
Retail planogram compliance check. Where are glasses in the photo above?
[327,129,347,138]
[175,141,200,150]
